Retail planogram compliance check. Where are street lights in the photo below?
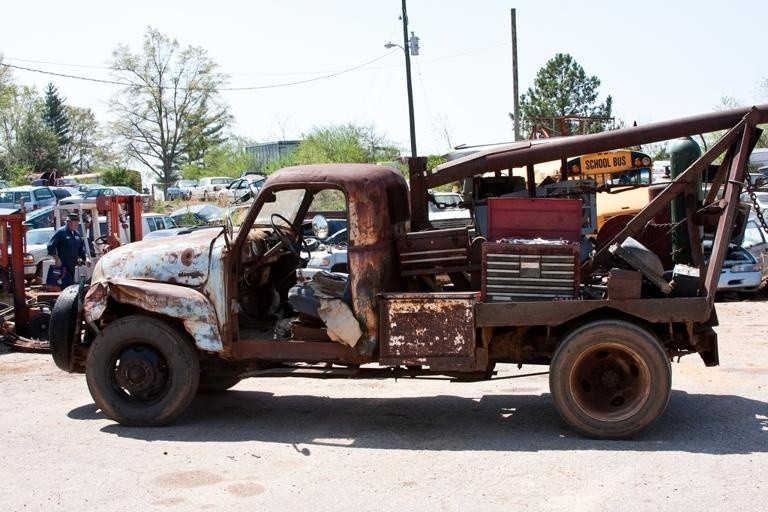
[384,0,420,157]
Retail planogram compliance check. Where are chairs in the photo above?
[288,270,351,316]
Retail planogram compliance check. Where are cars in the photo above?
[0,149,267,282]
[509,142,768,300]
[428,192,474,229]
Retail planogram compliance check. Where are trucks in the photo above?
[48,102,768,438]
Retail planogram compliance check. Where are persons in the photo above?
[47,212,92,290]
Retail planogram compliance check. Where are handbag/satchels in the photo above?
[46,265,66,286]
